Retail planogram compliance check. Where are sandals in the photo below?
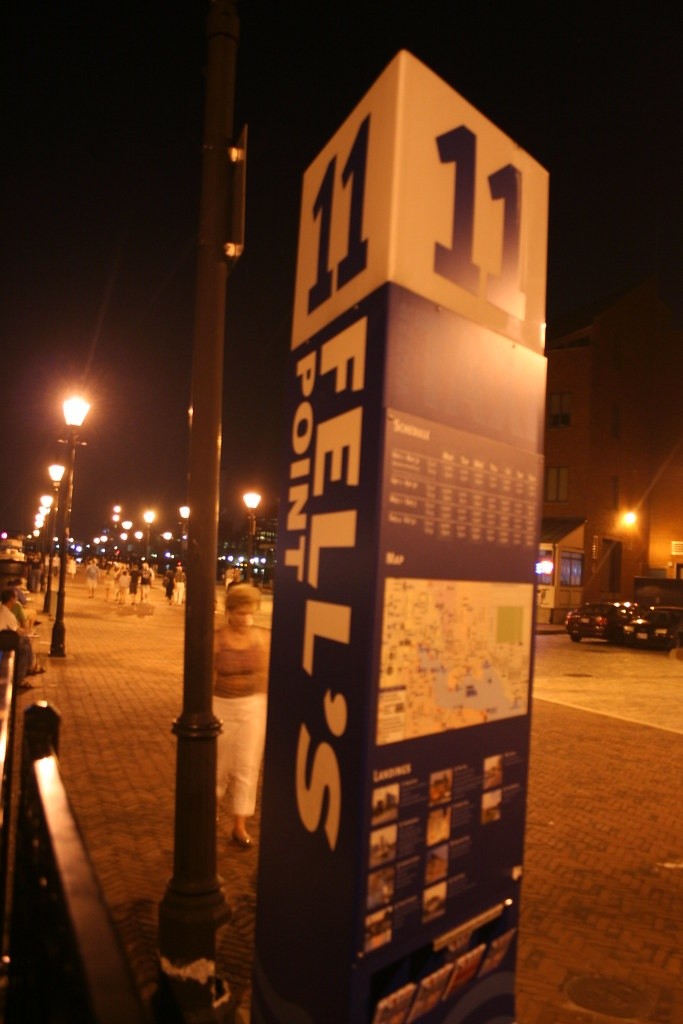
[16,679,34,689]
[26,664,46,676]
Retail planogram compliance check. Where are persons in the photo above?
[213,585,270,844]
[226,568,243,590]
[101,559,153,604]
[162,567,184,605]
[28,551,76,593]
[85,559,99,598]
[0,577,47,689]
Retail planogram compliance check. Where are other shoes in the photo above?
[27,631,39,637]
[233,830,255,849]
[34,621,42,626]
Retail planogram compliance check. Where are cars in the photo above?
[564,600,677,650]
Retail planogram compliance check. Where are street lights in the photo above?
[177,505,191,565]
[244,492,263,579]
[143,510,156,563]
[47,396,91,657]
[33,461,66,616]
